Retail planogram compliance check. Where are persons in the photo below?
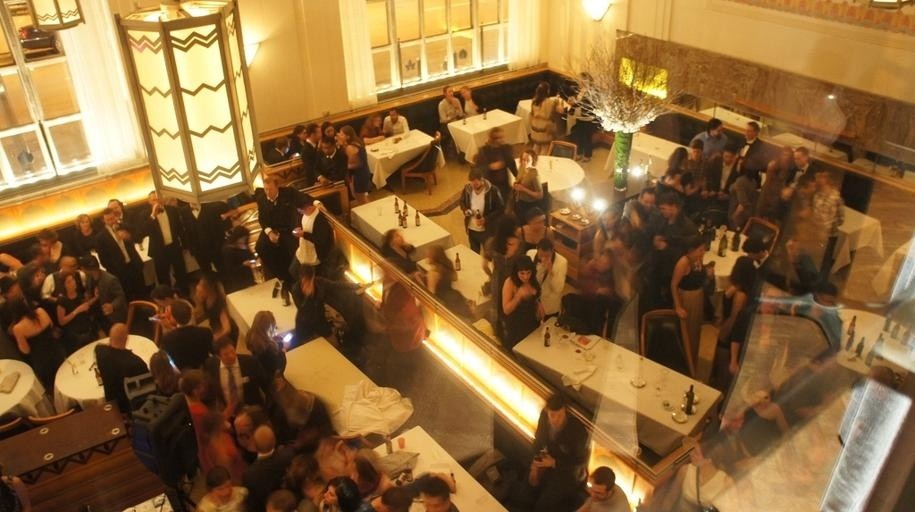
[265,106,410,216]
[1,190,232,392]
[94,278,462,512]
[222,184,474,389]
[439,74,844,512]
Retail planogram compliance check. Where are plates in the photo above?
[671,408,689,423]
[683,392,701,405]
[681,403,697,414]
[558,205,591,228]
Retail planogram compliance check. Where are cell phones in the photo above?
[294,153,300,156]
[468,212,474,216]
[283,333,295,344]
[292,230,297,234]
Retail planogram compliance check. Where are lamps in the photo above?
[580,2,611,22]
[113,0,268,204]
[237,13,268,67]
[27,0,87,33]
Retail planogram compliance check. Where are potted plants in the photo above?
[559,30,705,192]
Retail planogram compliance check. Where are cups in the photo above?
[398,436,405,449]
[370,139,379,151]
[378,204,383,217]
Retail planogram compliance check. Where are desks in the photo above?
[0,234,513,512]
[348,92,915,456]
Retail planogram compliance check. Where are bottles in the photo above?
[449,473,458,493]
[682,385,696,414]
[483,107,487,120]
[455,253,462,271]
[697,219,741,257]
[280,282,291,307]
[844,309,915,368]
[385,435,393,454]
[544,327,552,347]
[396,460,413,487]
[394,198,421,228]
[93,367,103,387]
[463,112,466,125]
